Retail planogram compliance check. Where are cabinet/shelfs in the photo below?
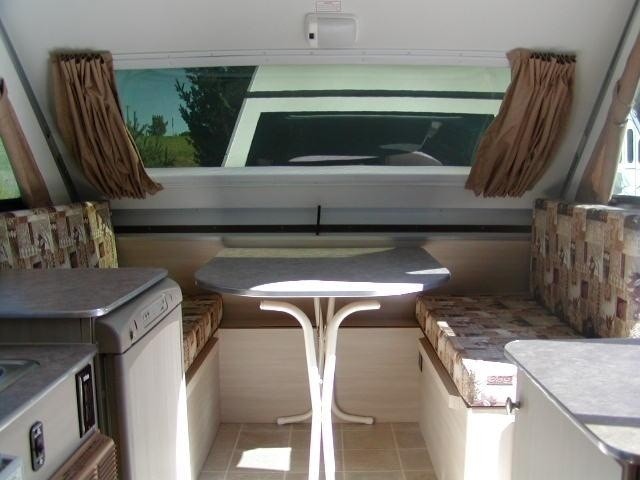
[502,337,640,480]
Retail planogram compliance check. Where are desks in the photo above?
[194,247,452,480]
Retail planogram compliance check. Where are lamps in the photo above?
[304,14,360,48]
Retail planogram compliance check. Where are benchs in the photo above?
[0,200,224,480]
[417,195,640,480]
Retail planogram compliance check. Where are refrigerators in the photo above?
[1,276,191,478]
